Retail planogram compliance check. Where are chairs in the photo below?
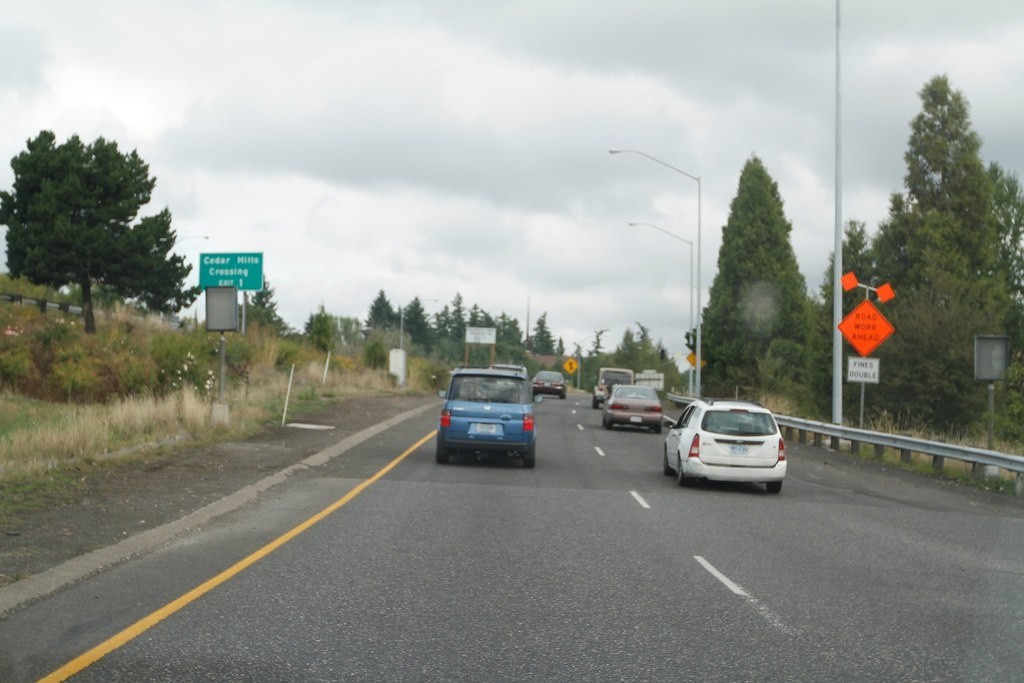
[619,391,627,397]
[739,423,752,431]
[498,388,517,403]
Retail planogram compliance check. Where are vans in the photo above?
[435,364,543,468]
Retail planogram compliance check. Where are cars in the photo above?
[531,371,567,399]
[601,384,664,433]
[663,397,788,493]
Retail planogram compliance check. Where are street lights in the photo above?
[609,149,701,401]
[627,221,693,398]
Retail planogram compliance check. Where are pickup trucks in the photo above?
[593,367,634,409]
